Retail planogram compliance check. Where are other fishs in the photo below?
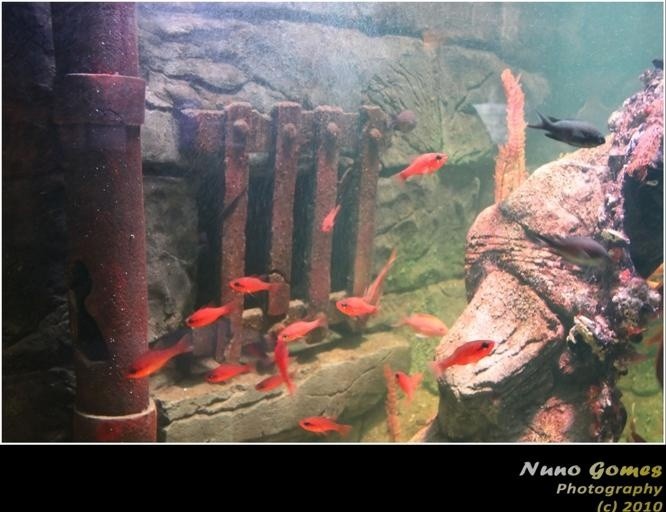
[185,244,450,397]
